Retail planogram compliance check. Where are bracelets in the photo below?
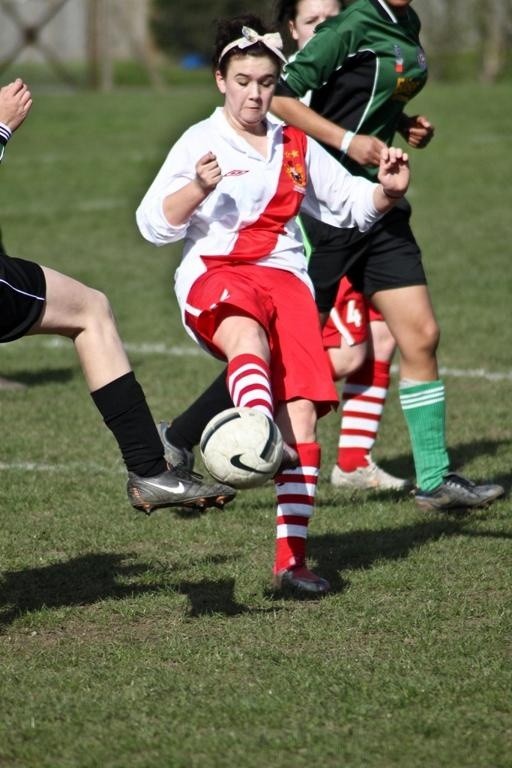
[340,131,356,155]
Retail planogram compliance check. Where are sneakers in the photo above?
[126,419,236,513]
[330,457,413,498]
[414,473,504,511]
[274,570,331,598]
[282,440,300,471]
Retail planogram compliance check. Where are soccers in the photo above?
[199,407,283,489]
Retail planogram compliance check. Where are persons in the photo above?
[135,16,410,593]
[0,77,236,510]
[289,0,406,489]
[156,0,506,511]
